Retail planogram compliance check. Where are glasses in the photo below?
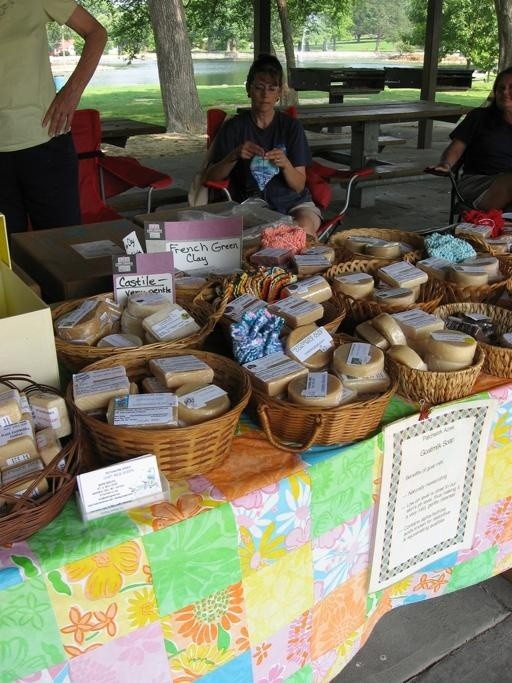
[248,83,279,94]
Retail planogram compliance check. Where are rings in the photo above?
[276,157,281,163]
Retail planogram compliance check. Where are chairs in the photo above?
[203,108,374,242]
[70,109,172,220]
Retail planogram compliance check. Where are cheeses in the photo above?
[0,221,512,508]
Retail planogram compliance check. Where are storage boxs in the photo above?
[0,260,61,393]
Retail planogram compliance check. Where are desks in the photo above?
[10,219,145,299]
[236,99,474,210]
[0,302,512,683]
[98,116,169,149]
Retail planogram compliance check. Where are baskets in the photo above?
[0,372,82,546]
[215,225,511,351]
[53,275,234,375]
[432,301,512,378]
[248,333,401,453]
[359,328,487,406]
[65,346,253,483]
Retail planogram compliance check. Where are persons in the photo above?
[423,67,512,212]
[201,54,325,234]
[0,0,109,234]
